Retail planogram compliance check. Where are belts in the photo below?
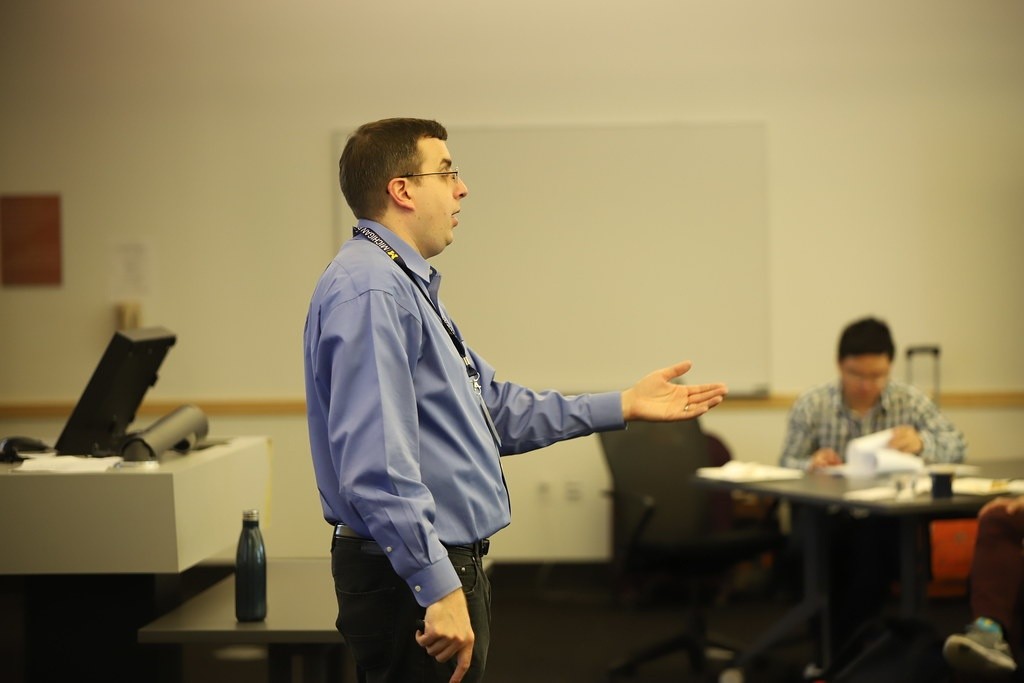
[334,524,489,557]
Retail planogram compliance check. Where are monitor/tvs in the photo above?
[54,325,177,458]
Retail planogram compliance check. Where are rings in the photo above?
[684,406,688,411]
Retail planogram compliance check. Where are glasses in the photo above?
[384,165,459,192]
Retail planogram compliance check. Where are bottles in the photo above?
[234,508,268,623]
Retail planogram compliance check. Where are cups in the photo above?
[927,471,956,498]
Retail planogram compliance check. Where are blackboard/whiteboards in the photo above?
[328,127,774,400]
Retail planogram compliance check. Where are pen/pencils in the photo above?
[416,619,458,674]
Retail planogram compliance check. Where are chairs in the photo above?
[591,411,781,682]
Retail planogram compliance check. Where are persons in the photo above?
[300,114,727,683]
[941,494,1024,678]
[779,314,965,673]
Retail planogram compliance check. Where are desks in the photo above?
[0,430,271,577]
[134,556,372,683]
[689,459,1022,683]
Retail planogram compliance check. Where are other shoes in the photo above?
[941,623,1017,676]
